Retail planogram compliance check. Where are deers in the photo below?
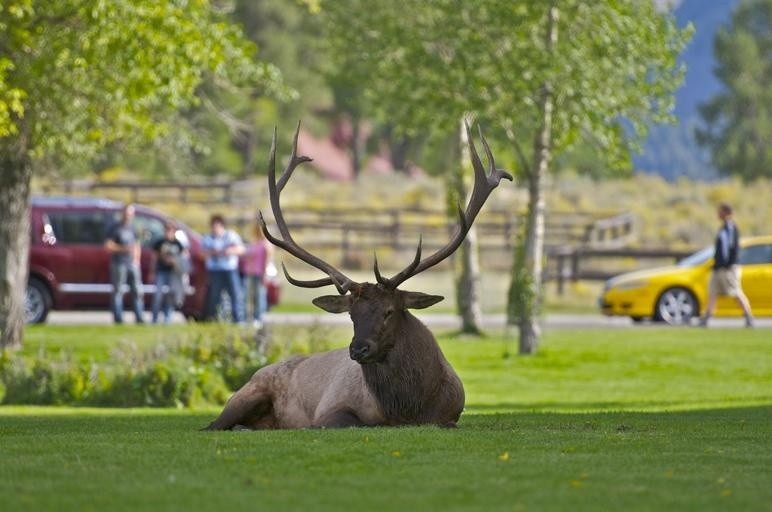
[196,115,515,432]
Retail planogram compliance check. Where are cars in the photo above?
[599,235,772,324]
[24,197,281,323]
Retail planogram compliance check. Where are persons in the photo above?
[689,199,759,328]
[149,219,192,325]
[197,212,246,323]
[240,219,273,332]
[102,201,145,323]
